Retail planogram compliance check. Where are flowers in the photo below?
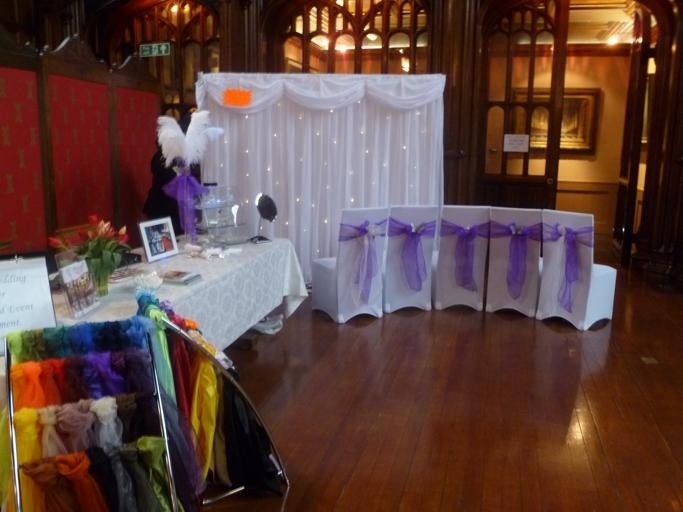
[48,213,131,293]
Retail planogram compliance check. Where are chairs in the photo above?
[487,206,537,324]
[542,210,615,328]
[433,204,486,319]
[315,206,386,322]
[386,206,433,322]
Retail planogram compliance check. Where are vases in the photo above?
[89,259,109,296]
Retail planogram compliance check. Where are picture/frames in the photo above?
[138,216,178,263]
[505,88,597,156]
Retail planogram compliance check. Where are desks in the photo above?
[49,234,286,353]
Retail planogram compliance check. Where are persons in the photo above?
[144,114,202,235]
